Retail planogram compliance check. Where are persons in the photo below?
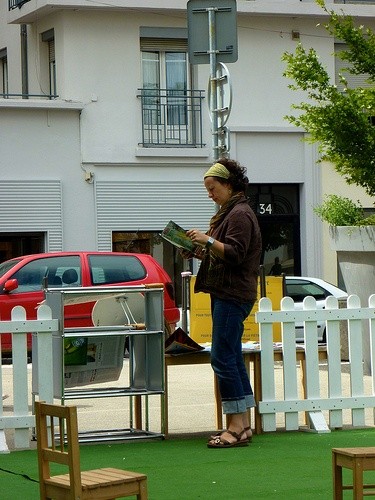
[268,256,282,277]
[178,157,261,449]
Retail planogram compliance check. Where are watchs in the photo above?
[205,237,215,247]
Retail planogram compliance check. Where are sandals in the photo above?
[207,430,249,448]
[208,427,252,443]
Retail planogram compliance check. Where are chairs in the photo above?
[33,400,148,500]
[332,447,375,500]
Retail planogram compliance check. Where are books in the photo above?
[159,220,206,258]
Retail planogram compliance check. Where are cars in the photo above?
[0,251,179,359]
[282,277,352,342]
